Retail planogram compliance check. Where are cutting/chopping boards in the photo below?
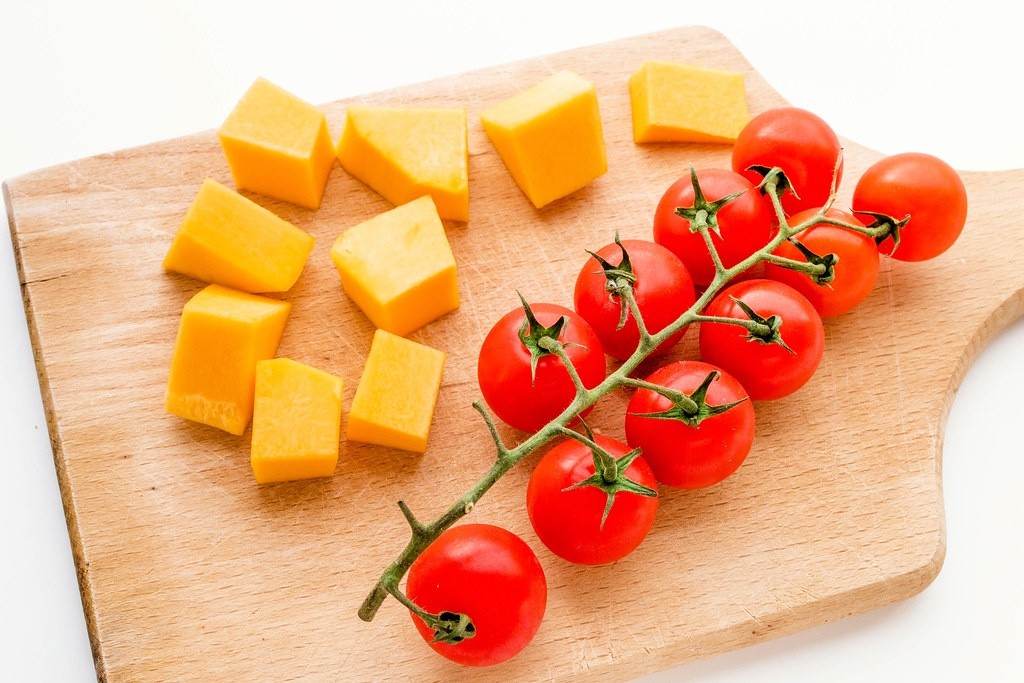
[2,26,1024,683]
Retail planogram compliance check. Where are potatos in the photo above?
[160,58,753,485]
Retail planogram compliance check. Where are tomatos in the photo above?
[479,105,968,565]
[405,523,548,667]
